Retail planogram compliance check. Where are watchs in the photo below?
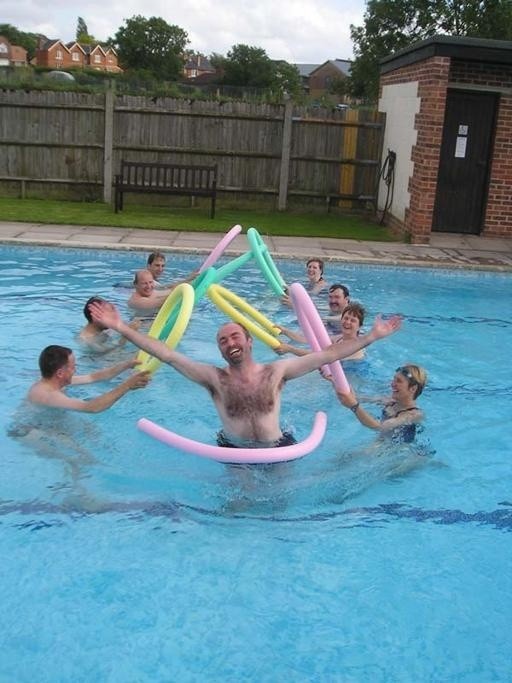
[351,402,359,412]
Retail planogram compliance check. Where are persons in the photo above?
[73,295,143,354]
[125,265,174,310]
[132,250,201,296]
[271,301,373,379]
[300,256,328,297]
[316,362,446,478]
[279,283,350,335]
[87,298,402,512]
[2,344,154,491]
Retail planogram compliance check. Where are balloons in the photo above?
[206,283,282,350]
[158,266,215,342]
[289,282,349,397]
[210,244,265,288]
[198,224,241,274]
[247,227,287,297]
[132,281,194,380]
[137,411,327,464]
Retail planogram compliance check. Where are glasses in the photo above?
[396,367,418,384]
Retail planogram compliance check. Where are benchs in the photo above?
[110,157,218,219]
